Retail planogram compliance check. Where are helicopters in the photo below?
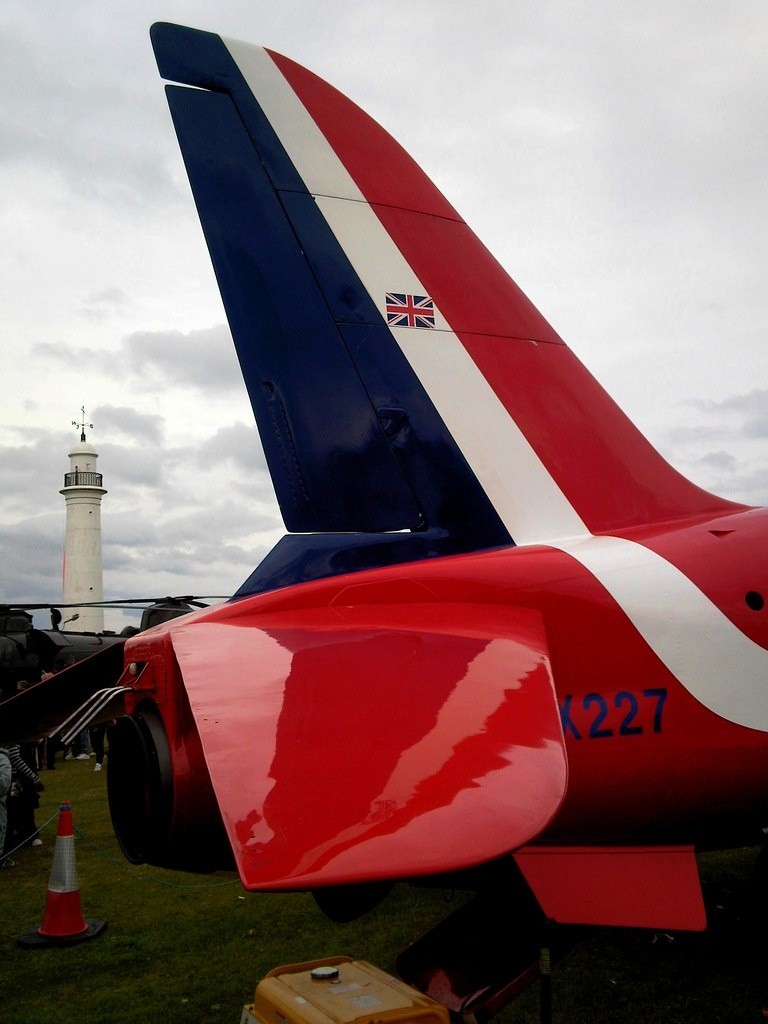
[0,594,234,704]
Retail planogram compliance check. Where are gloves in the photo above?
[35,782,45,791]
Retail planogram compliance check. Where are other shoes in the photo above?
[94,762,103,772]
[1,859,17,867]
[32,839,42,847]
[48,766,56,770]
[76,754,91,761]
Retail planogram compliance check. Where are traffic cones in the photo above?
[17,797,109,949]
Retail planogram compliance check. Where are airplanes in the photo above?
[1,20,767,927]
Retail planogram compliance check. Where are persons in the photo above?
[0,653,108,867]
[50,608,62,630]
